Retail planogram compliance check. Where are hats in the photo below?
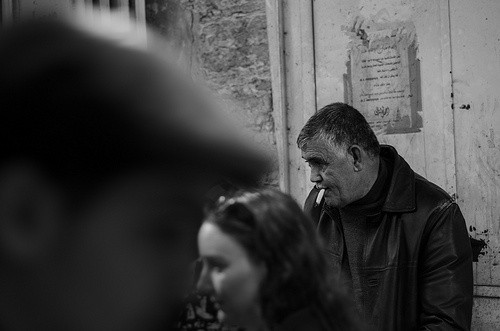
[1,14,271,182]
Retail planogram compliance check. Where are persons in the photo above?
[297,102,473,331]
[196,189,376,331]
